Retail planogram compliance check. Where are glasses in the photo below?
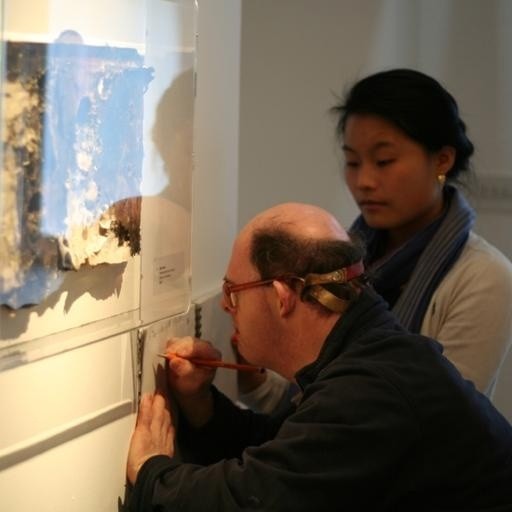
[223,274,306,308]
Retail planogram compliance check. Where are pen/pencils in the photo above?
[156,354,264,373]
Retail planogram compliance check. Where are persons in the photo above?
[125,201,511,510]
[231,67,511,420]
